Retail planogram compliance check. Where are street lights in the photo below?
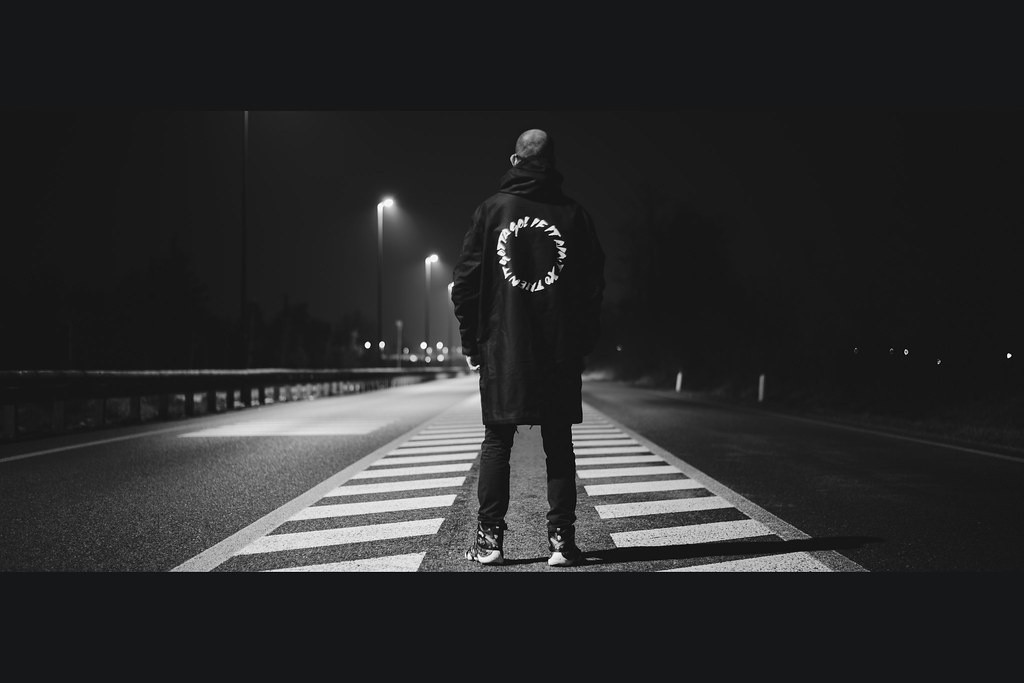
[375,198,396,361]
[396,319,403,368]
[424,253,439,347]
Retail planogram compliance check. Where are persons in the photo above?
[451,129,605,565]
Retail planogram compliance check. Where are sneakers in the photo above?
[547,526,587,566]
[465,521,504,564]
